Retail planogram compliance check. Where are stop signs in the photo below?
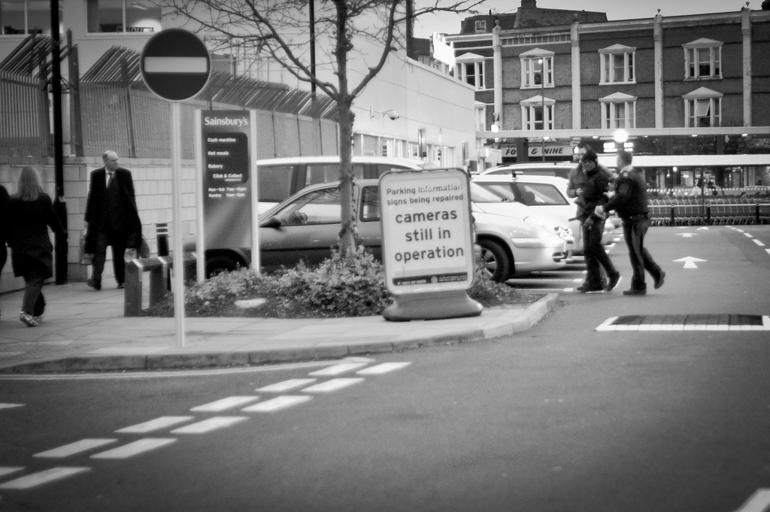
[139,28,211,101]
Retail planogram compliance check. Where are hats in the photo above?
[581,152,598,169]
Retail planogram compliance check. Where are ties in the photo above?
[108,173,112,187]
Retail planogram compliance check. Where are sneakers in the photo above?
[577,270,665,295]
[19,311,41,327]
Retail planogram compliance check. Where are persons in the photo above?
[565,142,607,290]
[1,184,14,277]
[593,150,666,296]
[82,149,142,290]
[577,149,620,292]
[3,164,68,328]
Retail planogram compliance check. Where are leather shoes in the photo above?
[118,283,124,288]
[88,280,101,290]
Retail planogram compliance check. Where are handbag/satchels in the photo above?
[80,235,94,265]
